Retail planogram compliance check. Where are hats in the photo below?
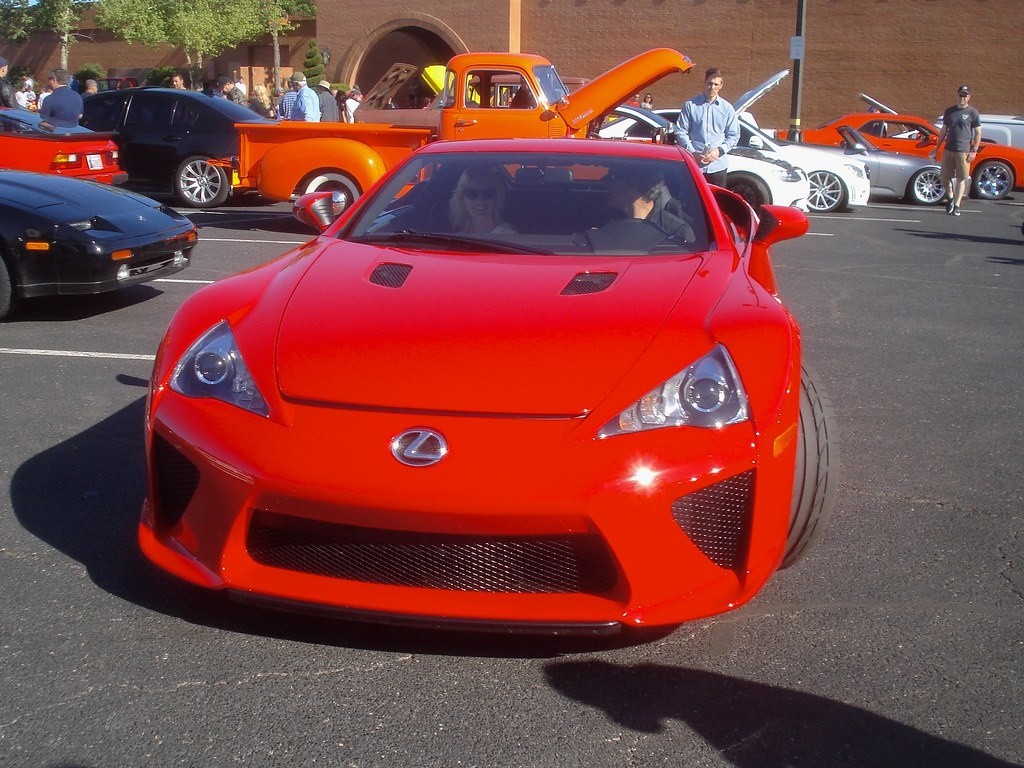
[0,56,8,66]
[217,75,228,90]
[316,80,331,93]
[290,71,308,81]
[958,86,971,94]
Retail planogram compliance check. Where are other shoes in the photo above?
[953,205,961,216]
[947,198,955,214]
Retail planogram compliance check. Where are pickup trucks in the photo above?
[205,46,698,233]
[351,63,610,131]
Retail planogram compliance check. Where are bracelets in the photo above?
[972,148,978,152]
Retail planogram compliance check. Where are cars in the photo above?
[422,63,616,124]
[599,108,872,213]
[893,113,1024,151]
[71,87,283,210]
[96,77,139,92]
[592,69,812,220]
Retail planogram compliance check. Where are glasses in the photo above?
[959,94,968,98]
[463,185,497,200]
[600,172,638,185]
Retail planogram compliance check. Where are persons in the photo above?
[581,163,697,245]
[384,97,398,108]
[447,165,521,235]
[0,58,98,127]
[420,94,439,110]
[867,107,889,137]
[166,68,364,123]
[504,77,653,114]
[929,84,982,216]
[674,67,741,188]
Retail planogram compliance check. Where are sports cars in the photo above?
[136,138,836,640]
[772,124,972,206]
[776,113,1024,200]
[0,166,198,319]
[0,104,131,191]
